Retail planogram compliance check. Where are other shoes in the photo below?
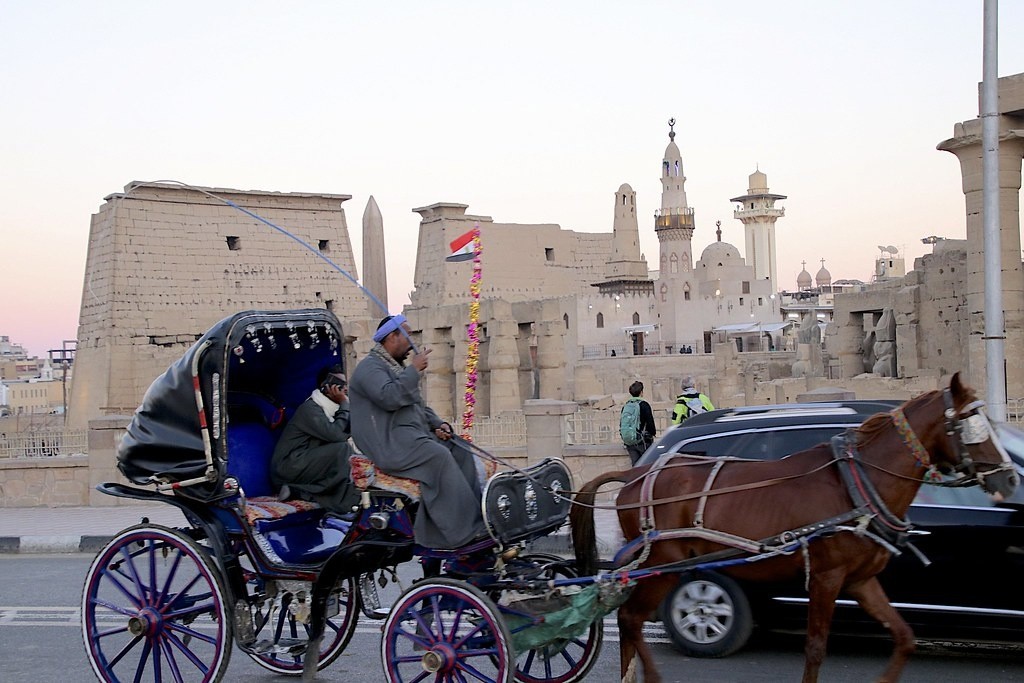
[278,485,291,501]
[447,551,541,580]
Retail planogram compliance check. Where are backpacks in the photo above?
[678,392,707,417]
[621,399,650,446]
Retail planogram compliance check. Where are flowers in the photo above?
[460,223,482,442]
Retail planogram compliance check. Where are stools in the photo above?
[348,437,420,507]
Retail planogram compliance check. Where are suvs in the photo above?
[629,398,1024,661]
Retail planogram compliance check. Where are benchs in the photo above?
[210,422,325,532]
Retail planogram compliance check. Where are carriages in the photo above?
[79,305,1022,683]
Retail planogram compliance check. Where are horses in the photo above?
[570,371,1022,683]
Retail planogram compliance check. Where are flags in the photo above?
[450,229,477,256]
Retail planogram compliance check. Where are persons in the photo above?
[671,376,715,425]
[348,315,519,569]
[644,348,656,355]
[610,350,616,356]
[771,345,775,351]
[618,381,656,468]
[680,345,692,354]
[272,364,371,516]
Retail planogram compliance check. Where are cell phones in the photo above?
[324,374,346,391]
